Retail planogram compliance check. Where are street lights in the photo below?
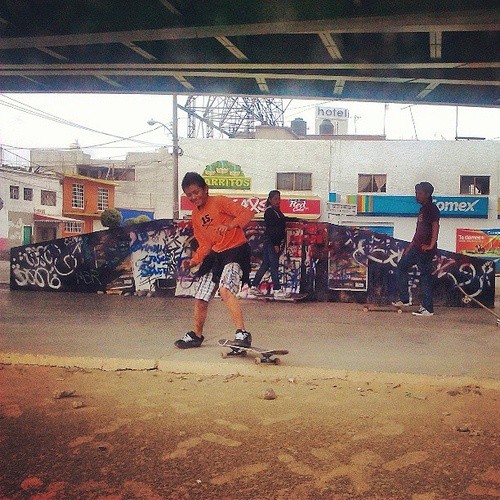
[147,117,173,137]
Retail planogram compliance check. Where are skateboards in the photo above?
[362,304,409,313]
[457,284,500,326]
[217,338,290,364]
[248,292,307,304]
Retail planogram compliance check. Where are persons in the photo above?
[247,190,306,298]
[174,171,252,349]
[391,182,440,316]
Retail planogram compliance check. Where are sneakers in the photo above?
[412,305,434,317]
[232,329,252,348]
[392,299,412,308]
[246,285,262,296]
[174,331,204,348]
[274,290,291,298]
